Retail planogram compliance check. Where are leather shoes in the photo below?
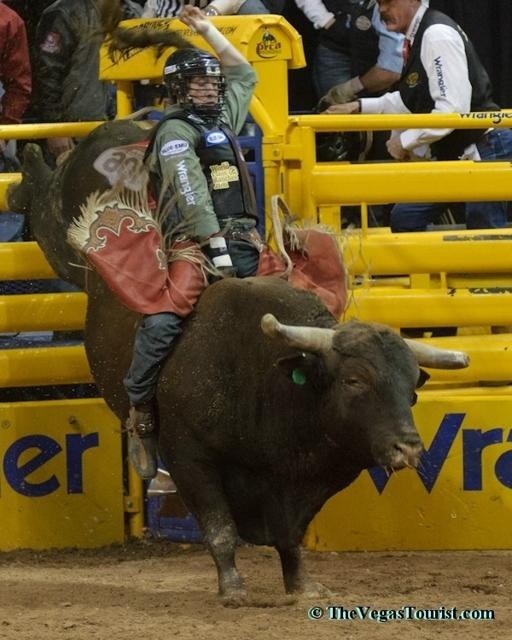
[129,401,159,478]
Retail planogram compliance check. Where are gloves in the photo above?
[316,75,369,113]
[200,237,233,285]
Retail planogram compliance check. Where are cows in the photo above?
[6,0,469,609]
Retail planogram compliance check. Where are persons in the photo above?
[0,0,512,480]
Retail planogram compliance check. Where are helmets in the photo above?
[163,48,228,118]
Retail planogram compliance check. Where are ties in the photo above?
[403,38,411,65]
